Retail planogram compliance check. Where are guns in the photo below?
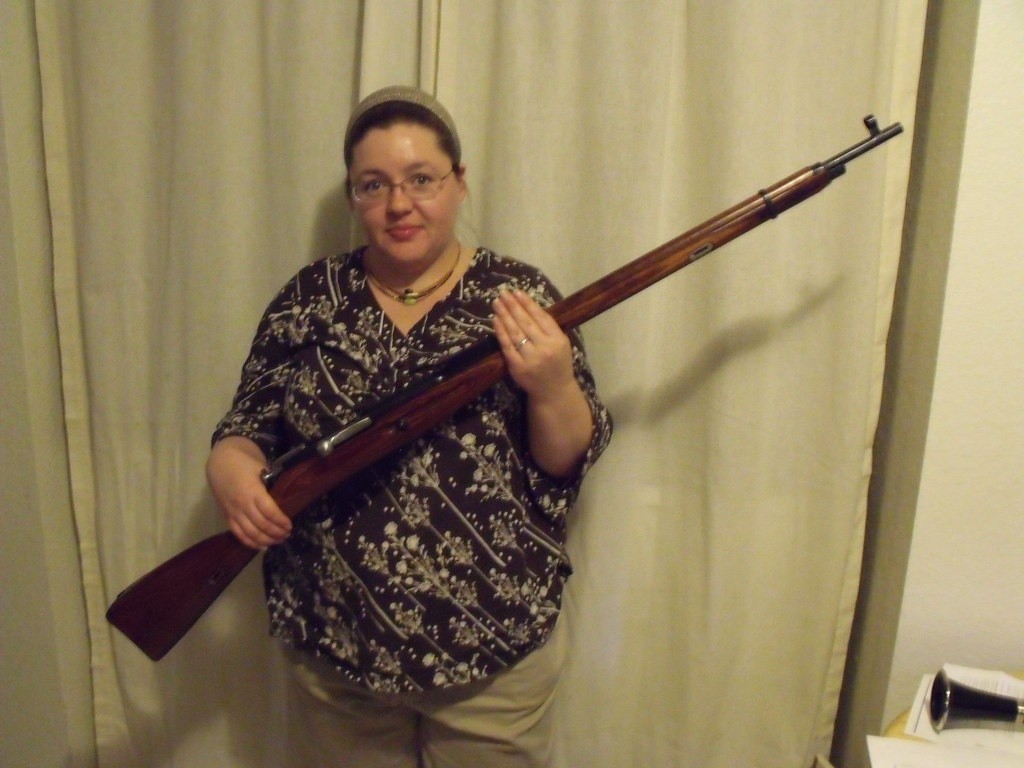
[106,114,905,661]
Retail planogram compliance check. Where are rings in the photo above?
[515,338,529,350]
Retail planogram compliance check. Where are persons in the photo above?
[205,85,614,768]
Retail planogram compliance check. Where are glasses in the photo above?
[347,164,456,208]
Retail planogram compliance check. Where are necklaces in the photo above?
[367,240,461,304]
